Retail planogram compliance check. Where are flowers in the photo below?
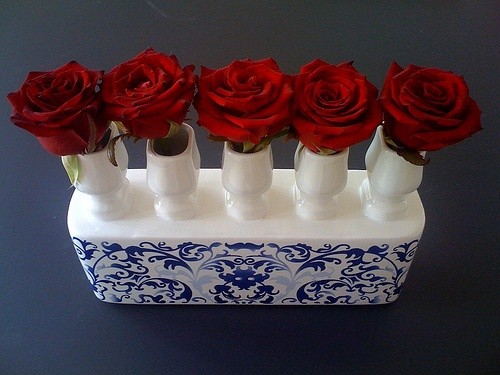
[101,46,198,144]
[289,54,381,157]
[378,61,482,168]
[191,57,294,152]
[6,57,109,190]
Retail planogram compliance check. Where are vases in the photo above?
[62,117,428,308]
